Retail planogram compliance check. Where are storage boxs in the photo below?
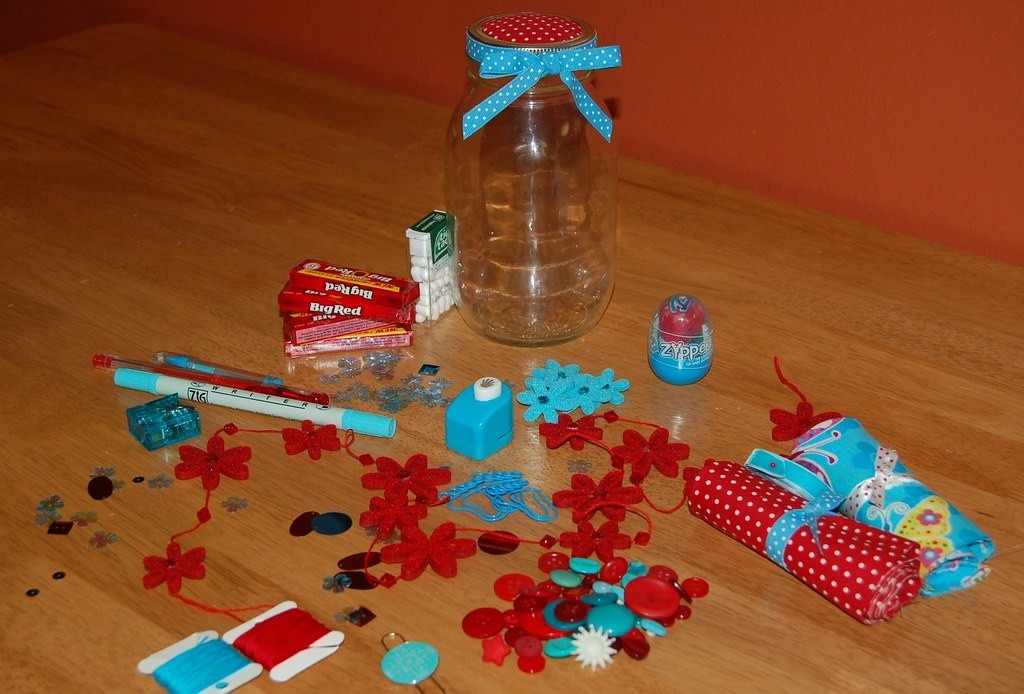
[277,258,420,358]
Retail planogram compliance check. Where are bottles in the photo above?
[443,12,618,345]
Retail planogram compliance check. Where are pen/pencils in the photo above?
[113,368,397,439]
[93,353,331,406]
[151,350,283,384]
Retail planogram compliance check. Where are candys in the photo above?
[410,255,454,323]
